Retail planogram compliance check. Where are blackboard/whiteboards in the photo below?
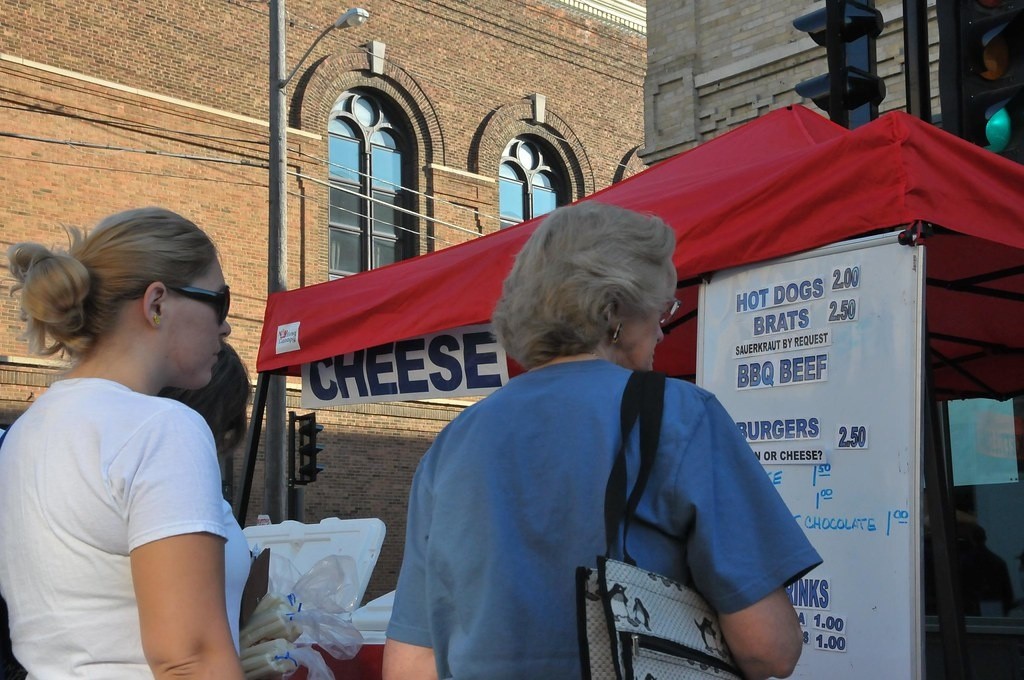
[696,228,926,680]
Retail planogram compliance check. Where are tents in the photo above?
[234,103,1024,680]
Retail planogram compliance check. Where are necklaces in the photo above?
[585,352,598,359]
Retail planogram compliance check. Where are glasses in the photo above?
[659,295,682,326]
[119,280,230,326]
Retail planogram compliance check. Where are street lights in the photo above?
[264,6,371,529]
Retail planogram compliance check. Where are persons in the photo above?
[381,197,824,680]
[921,486,1014,619]
[0,206,253,680]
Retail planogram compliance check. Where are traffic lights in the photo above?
[938,0,1023,163]
[793,0,885,115]
[298,411,324,482]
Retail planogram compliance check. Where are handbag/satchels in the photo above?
[575,369,747,680]
